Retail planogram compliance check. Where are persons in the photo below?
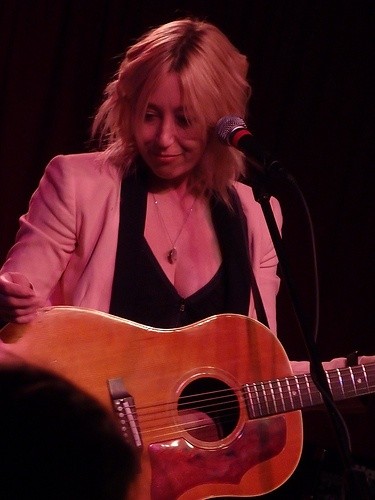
[1,20,374,375]
[0,363,142,500]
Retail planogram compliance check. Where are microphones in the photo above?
[215,115,287,177]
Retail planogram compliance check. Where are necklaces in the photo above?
[148,185,203,264]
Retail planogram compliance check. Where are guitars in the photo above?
[0,305,375,500]
